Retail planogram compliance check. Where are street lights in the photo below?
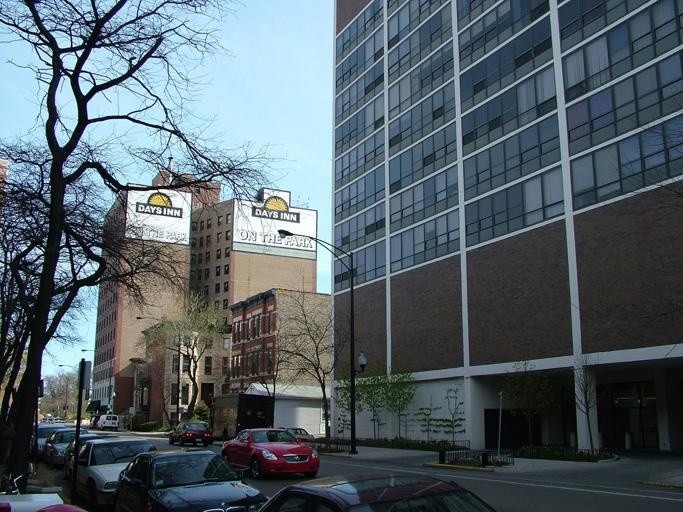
[137,316,181,425]
[82,349,112,415]
[59,364,79,372]
[277,230,366,454]
